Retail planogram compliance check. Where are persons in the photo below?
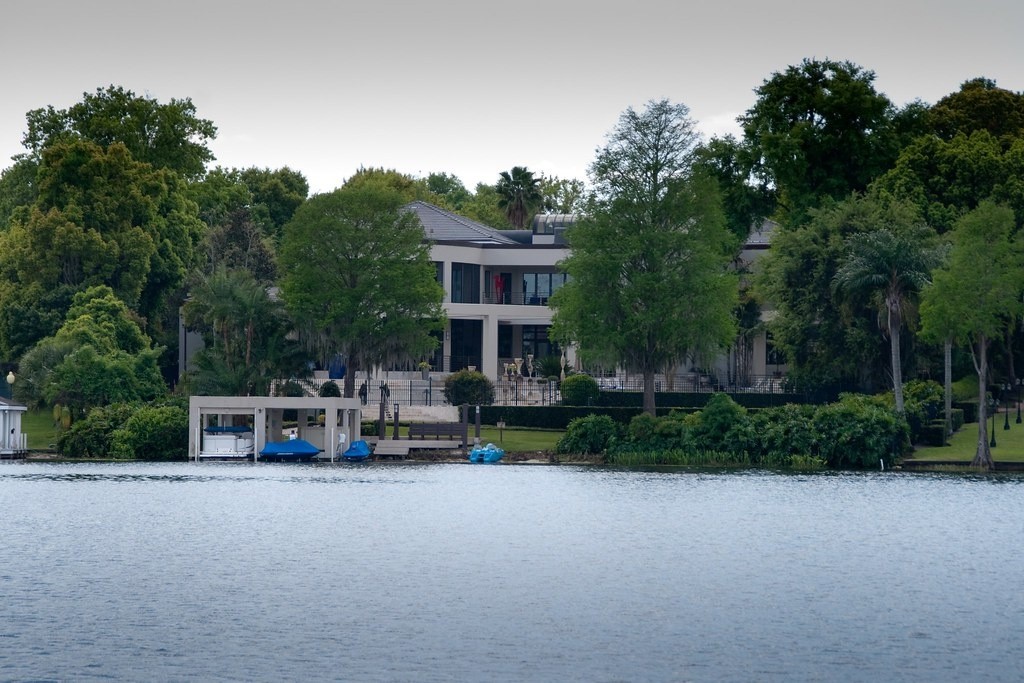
[358,383,367,407]
[385,383,390,404]
[318,412,325,423]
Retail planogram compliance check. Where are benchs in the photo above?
[407,421,463,440]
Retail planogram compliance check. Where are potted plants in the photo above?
[419,362,432,380]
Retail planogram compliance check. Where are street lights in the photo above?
[7,371,15,449]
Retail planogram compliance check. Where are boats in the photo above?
[343,440,372,462]
[199,425,255,461]
[259,439,320,460]
[470,443,505,464]
